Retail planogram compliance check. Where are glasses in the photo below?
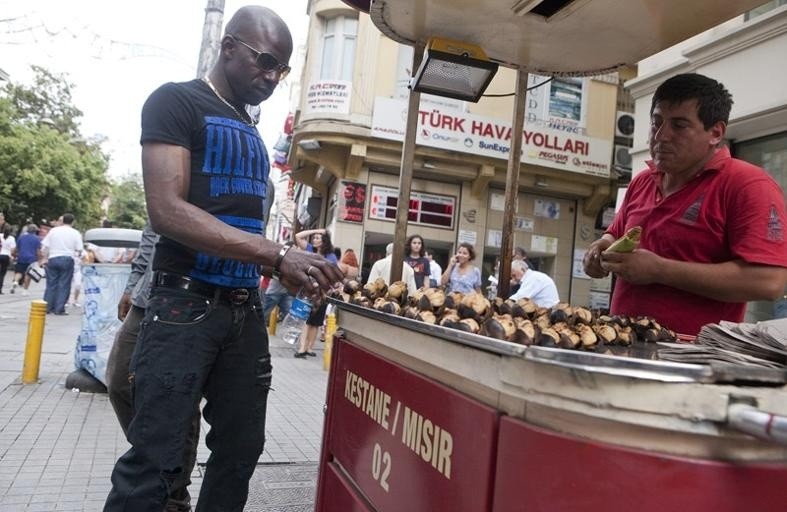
[238,40,291,80]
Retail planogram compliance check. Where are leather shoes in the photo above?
[295,350,316,357]
[49,310,68,315]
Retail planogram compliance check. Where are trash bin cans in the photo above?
[65,226,144,394]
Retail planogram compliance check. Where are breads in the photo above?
[331,276,678,350]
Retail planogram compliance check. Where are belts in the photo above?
[153,270,258,305]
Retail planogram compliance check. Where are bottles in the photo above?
[279,287,314,346]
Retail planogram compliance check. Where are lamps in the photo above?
[296,139,320,149]
[408,35,498,104]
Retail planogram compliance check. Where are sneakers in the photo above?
[10,281,29,296]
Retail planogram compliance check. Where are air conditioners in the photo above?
[614,109,634,138]
[613,144,633,167]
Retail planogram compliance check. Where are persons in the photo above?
[581,73,787,336]
[103,216,204,512]
[258,227,561,358]
[0,212,138,316]
[101,4,349,511]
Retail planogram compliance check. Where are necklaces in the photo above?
[204,73,256,128]
